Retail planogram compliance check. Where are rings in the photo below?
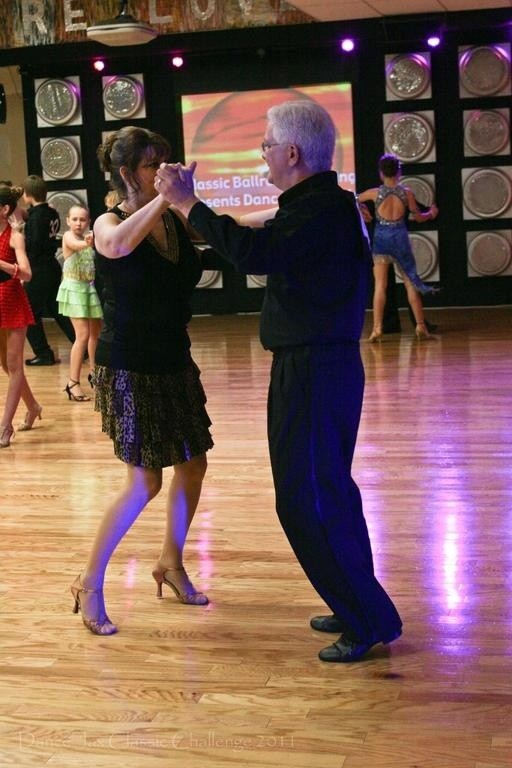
[158,178,162,184]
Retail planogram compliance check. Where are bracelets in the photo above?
[430,211,435,217]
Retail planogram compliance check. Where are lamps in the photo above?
[85,1,159,50]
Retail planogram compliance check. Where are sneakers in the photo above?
[382,323,400,333]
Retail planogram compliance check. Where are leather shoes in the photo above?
[318,624,403,664]
[83,350,89,361]
[25,356,55,366]
[309,614,344,634]
[413,323,439,333]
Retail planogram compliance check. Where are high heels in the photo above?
[18,404,43,431]
[367,324,383,344]
[71,574,119,636]
[63,378,90,401]
[88,372,95,389]
[152,560,210,606]
[1,424,15,448]
[415,321,437,342]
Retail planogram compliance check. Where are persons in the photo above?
[70,125,372,635]
[154,100,401,663]
[357,153,437,342]
[0,175,122,448]
[384,198,437,333]
